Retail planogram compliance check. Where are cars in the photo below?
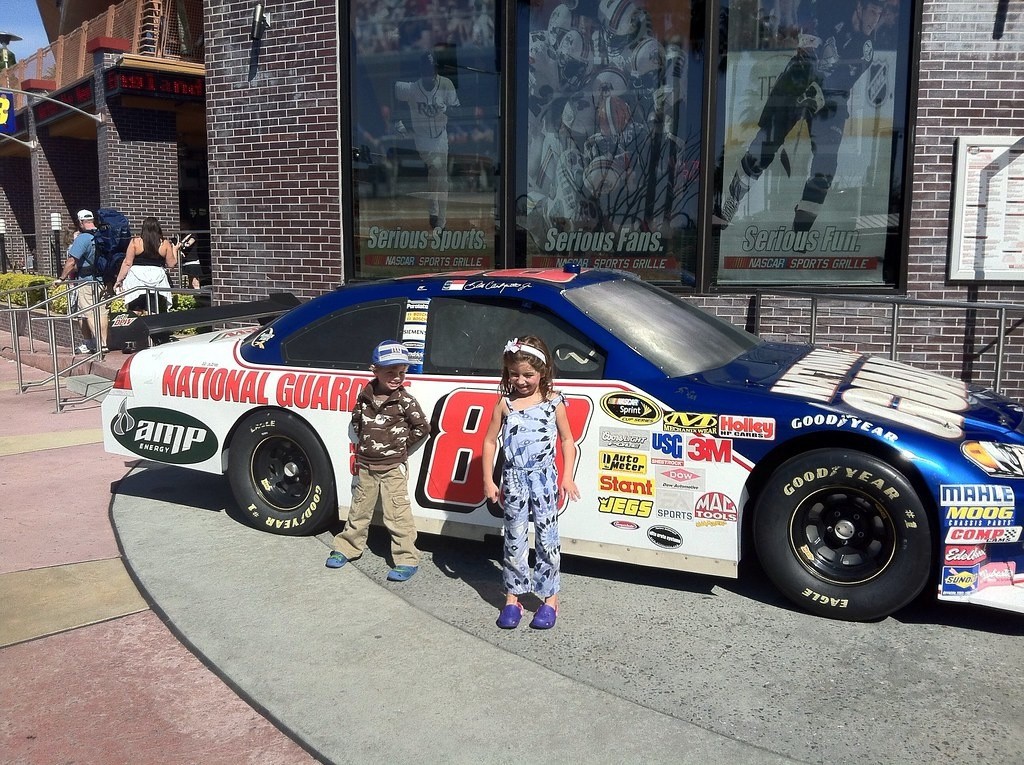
[97,263,1024,620]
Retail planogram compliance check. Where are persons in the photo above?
[180,211,204,297]
[527,0,686,252]
[113,217,182,353]
[326,340,430,580]
[724,0,885,253]
[483,336,581,629]
[63,227,81,312]
[52,210,111,353]
[352,0,499,239]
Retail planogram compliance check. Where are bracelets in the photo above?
[59,277,64,281]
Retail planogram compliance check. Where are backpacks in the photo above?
[75,207,131,281]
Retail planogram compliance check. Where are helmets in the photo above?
[534,0,691,201]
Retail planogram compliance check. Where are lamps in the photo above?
[251,4,271,42]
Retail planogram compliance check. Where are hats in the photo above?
[76,208,95,219]
[373,340,414,366]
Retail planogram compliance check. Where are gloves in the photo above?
[796,83,828,121]
[794,32,828,79]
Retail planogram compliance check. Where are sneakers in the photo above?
[721,198,742,231]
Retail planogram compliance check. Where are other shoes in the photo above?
[326,550,349,568]
[91,345,110,354]
[74,340,95,354]
[430,215,449,241]
[386,565,418,581]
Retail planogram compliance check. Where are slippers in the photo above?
[530,603,558,630]
[496,602,524,628]
